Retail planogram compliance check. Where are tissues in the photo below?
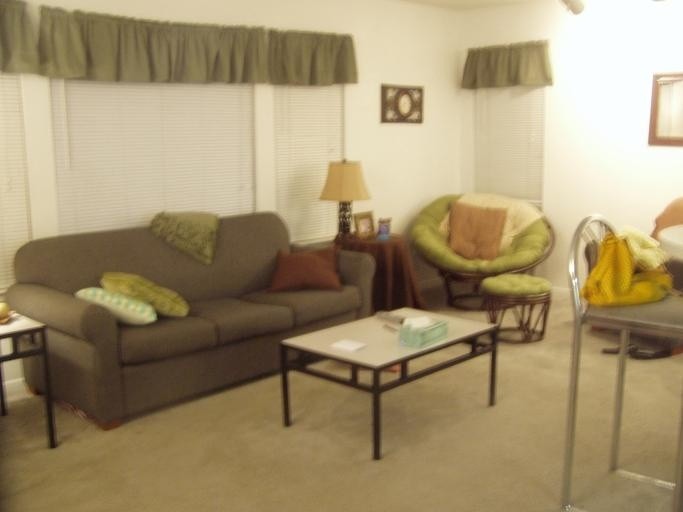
[399,315,449,349]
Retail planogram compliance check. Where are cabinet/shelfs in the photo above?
[561,214,682,510]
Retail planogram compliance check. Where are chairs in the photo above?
[410,192,556,311]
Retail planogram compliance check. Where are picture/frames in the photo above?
[646,72,682,150]
[353,211,375,240]
[375,82,424,125]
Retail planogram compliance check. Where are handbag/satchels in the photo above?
[580,229,673,308]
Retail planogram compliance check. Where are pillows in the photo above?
[97,271,191,322]
[447,199,508,259]
[268,244,343,295]
[71,285,158,327]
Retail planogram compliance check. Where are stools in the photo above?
[480,273,552,344]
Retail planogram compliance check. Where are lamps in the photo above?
[316,158,372,242]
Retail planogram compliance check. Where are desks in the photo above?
[336,232,425,314]
[0,301,61,451]
[277,304,501,461]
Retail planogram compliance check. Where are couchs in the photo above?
[1,208,374,430]
